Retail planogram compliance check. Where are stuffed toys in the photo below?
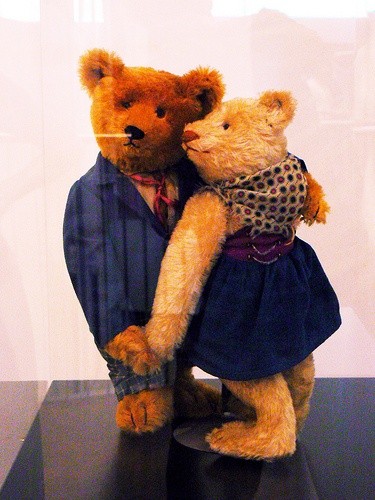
[65,47,225,432]
[147,88,342,459]
